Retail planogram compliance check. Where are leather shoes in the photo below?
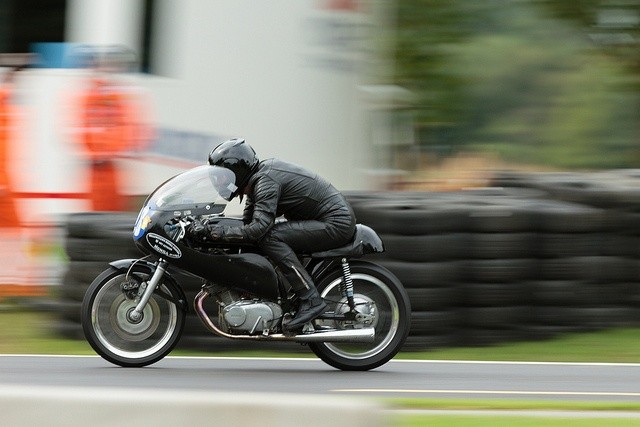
[284,296,330,327]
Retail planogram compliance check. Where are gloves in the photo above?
[186,220,208,241]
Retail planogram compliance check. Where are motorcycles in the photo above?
[81,165,411,371]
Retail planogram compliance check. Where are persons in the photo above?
[188,138,357,330]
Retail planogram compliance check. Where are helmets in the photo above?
[207,137,259,202]
